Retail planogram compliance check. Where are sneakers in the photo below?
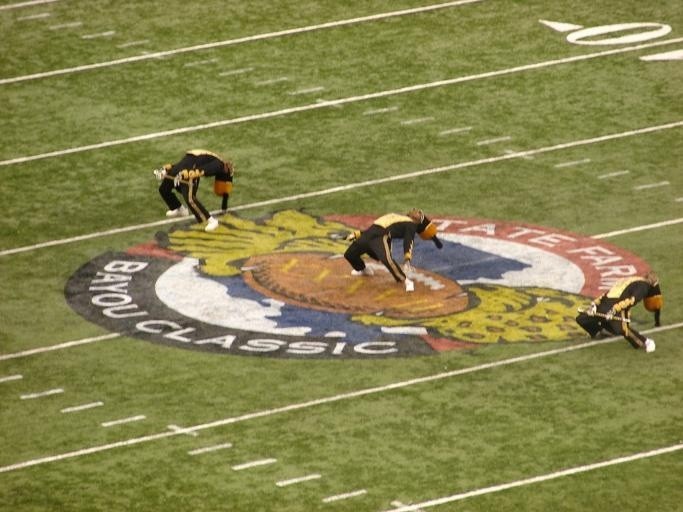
[351,267,375,276]
[645,338,655,353]
[204,216,218,232]
[405,280,414,292]
[165,205,189,217]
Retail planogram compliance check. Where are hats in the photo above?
[417,215,438,241]
[644,281,663,312]
[214,173,233,196]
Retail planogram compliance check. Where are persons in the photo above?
[153,149,234,233]
[575,271,663,353]
[342,206,442,293]
